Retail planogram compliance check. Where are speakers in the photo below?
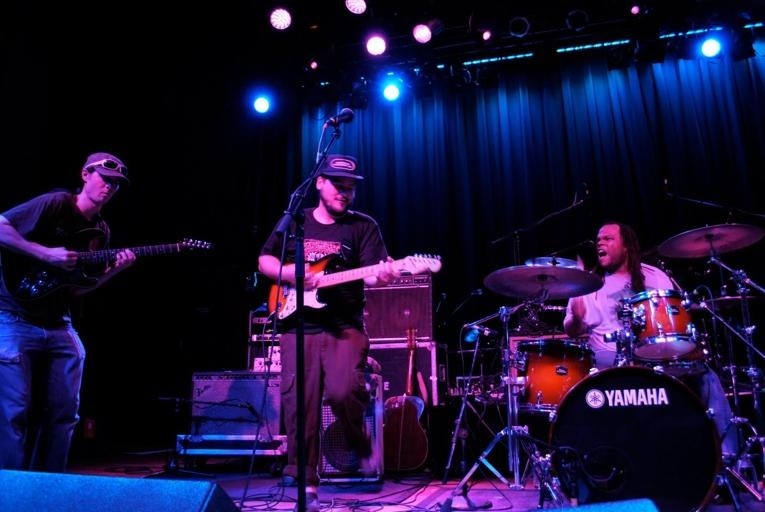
[0,467,241,512]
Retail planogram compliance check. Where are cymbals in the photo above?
[526,257,580,269]
[705,294,765,311]
[484,266,603,300]
[658,223,764,259]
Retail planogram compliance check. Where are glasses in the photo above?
[85,159,128,176]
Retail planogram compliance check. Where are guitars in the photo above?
[268,251,441,334]
[0,226,210,320]
[383,331,429,471]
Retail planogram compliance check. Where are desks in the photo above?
[508,331,591,492]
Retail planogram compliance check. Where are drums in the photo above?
[549,364,721,512]
[652,330,709,377]
[517,339,595,413]
[628,289,696,362]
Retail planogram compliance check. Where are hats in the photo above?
[83,152,128,180]
[322,155,364,180]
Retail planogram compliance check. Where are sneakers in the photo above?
[293,485,320,512]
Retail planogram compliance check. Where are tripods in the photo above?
[719,419,765,511]
[728,438,764,475]
[443,423,571,510]
[693,474,743,511]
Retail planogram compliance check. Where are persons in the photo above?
[560,219,741,460]
[256,150,402,511]
[0,149,138,472]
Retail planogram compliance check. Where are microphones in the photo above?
[327,110,354,127]
[584,239,595,246]
[578,175,592,200]
